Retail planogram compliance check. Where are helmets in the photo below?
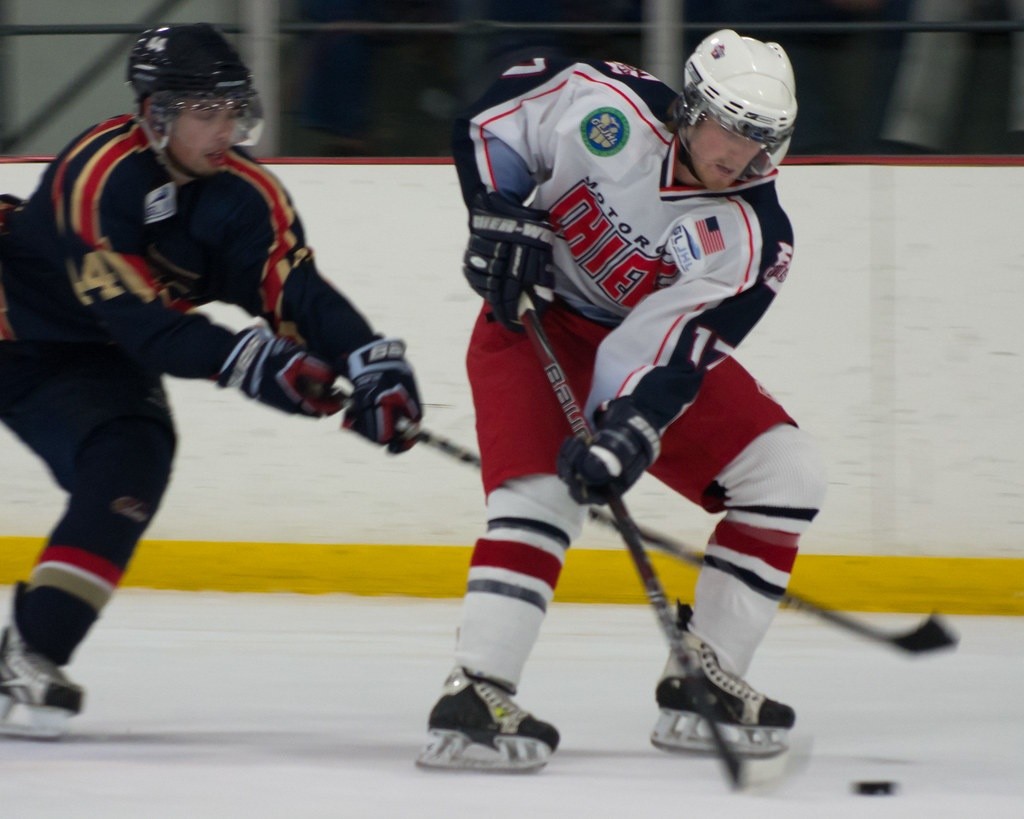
[672,29,799,182]
[126,21,264,151]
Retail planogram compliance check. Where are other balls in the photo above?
[849,776,898,799]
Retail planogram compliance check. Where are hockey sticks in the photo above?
[320,380,960,658]
[510,283,814,795]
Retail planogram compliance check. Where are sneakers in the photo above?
[412,664,559,767]
[1,580,84,741]
[652,597,795,758]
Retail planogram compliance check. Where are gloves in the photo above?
[463,194,554,335]
[218,324,355,420]
[343,338,422,453]
[557,396,660,506]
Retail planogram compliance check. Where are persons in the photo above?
[411,27,832,781]
[0,17,427,748]
